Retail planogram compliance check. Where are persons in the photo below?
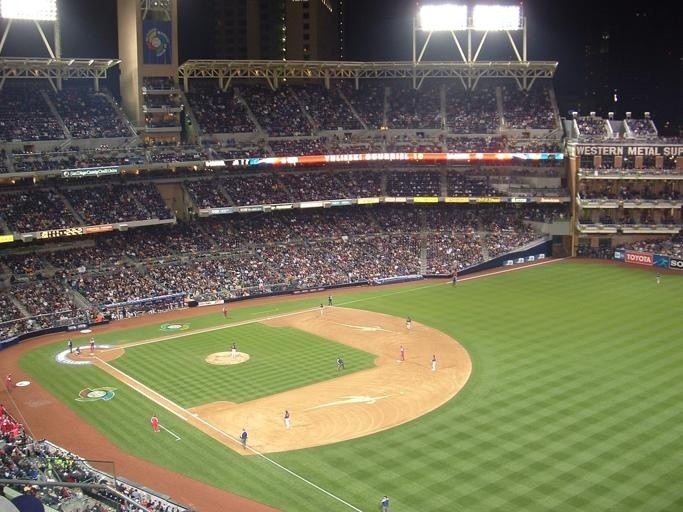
[404,315,412,329]
[229,341,237,358]
[335,356,345,371]
[0,76,190,347]
[239,427,247,449]
[654,271,663,286]
[283,409,290,428]
[430,353,437,371]
[318,301,324,317]
[326,295,333,305]
[74,345,80,356]
[221,303,228,318]
[66,337,73,354]
[379,494,391,512]
[2,373,11,393]
[0,405,188,510]
[451,267,458,286]
[423,89,682,278]
[398,344,405,362]
[190,80,422,298]
[88,335,95,354]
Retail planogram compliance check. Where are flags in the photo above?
[139,17,174,69]
